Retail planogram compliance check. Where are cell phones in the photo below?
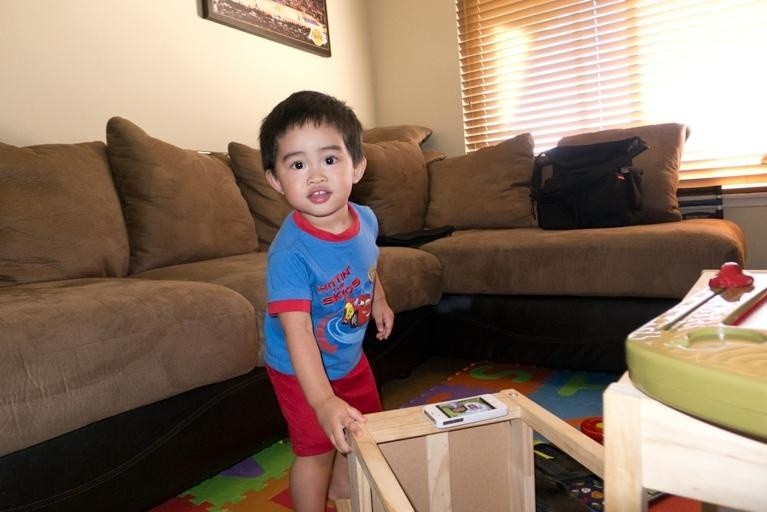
[424,392,509,430]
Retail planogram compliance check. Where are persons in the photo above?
[257,91,395,512]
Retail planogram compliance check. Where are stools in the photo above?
[603,370,767,512]
[345,388,605,512]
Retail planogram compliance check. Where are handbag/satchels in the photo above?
[529,137,650,230]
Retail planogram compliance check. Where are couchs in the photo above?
[0,117,745,512]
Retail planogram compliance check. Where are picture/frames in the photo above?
[202,0,332,57]
